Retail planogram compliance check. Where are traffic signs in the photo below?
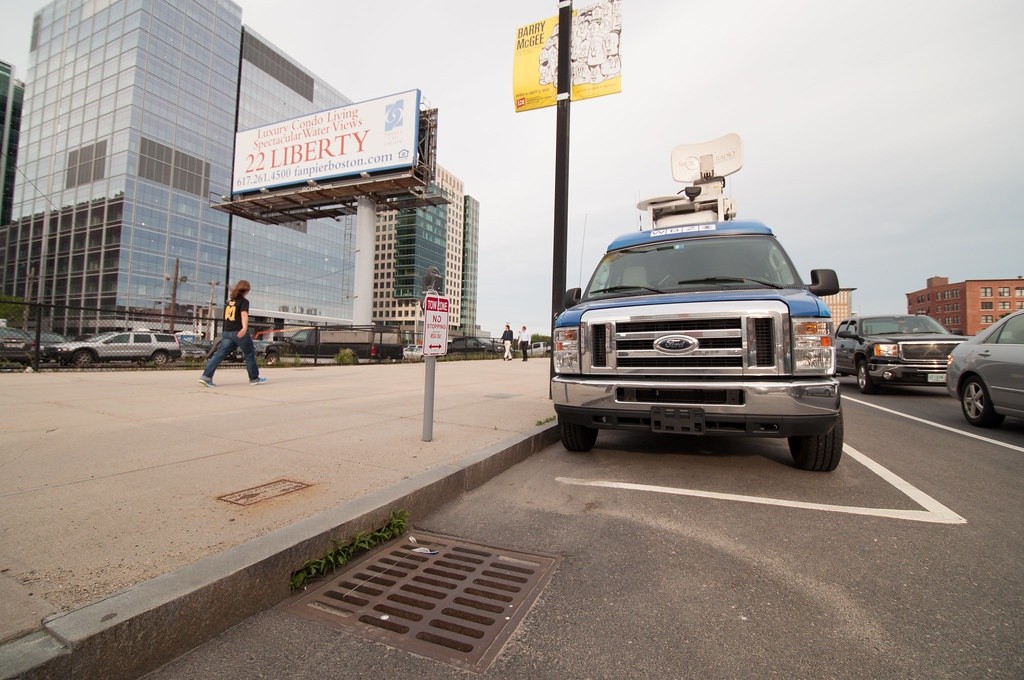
[423,294,448,356]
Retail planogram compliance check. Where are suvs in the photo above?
[553,135,844,471]
[46,331,181,368]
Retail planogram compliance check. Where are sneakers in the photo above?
[252,377,269,385]
[199,375,215,387]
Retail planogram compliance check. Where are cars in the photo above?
[403,343,424,360]
[1,324,265,373]
[946,309,1024,426]
[447,337,513,352]
[835,313,972,395]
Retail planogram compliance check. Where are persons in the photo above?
[198,279,267,389]
[518,326,531,361]
[500,325,513,361]
[370,346,377,358]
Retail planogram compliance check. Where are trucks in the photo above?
[290,329,402,359]
[521,342,552,356]
